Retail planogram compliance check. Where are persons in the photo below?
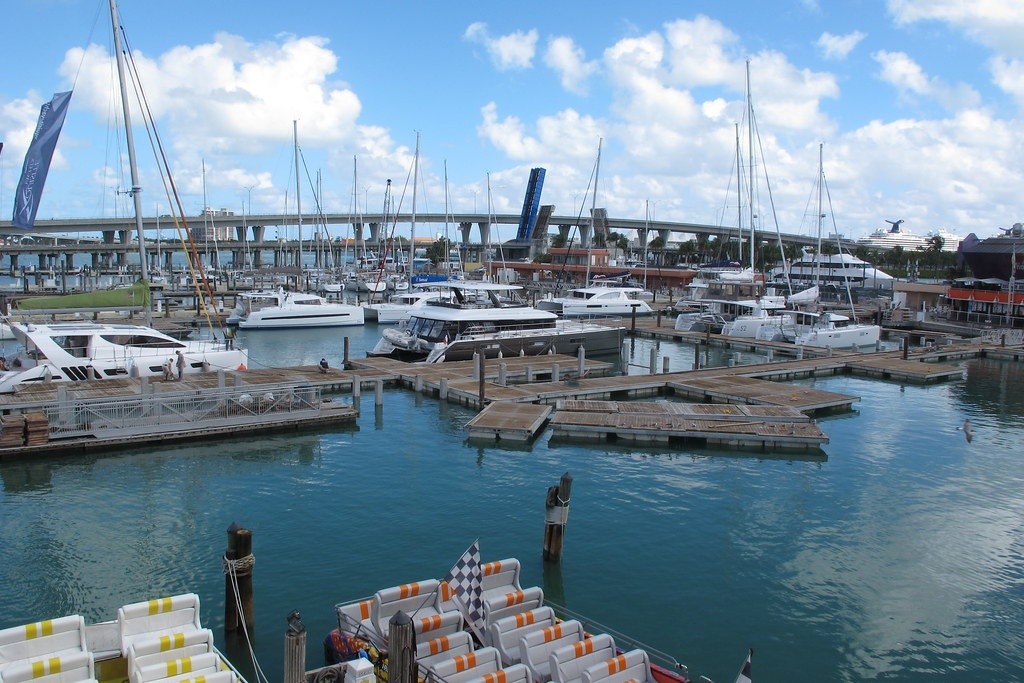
[318,358,330,373]
[224,323,236,351]
[175,350,186,382]
[162,358,178,381]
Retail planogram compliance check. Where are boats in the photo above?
[767,244,894,290]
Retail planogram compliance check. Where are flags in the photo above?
[444,538,486,637]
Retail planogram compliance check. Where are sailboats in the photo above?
[0,0,249,399]
[303,60,895,357]
[226,119,364,327]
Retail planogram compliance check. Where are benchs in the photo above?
[118,592,240,683]
[0,614,98,683]
[339,558,658,683]
